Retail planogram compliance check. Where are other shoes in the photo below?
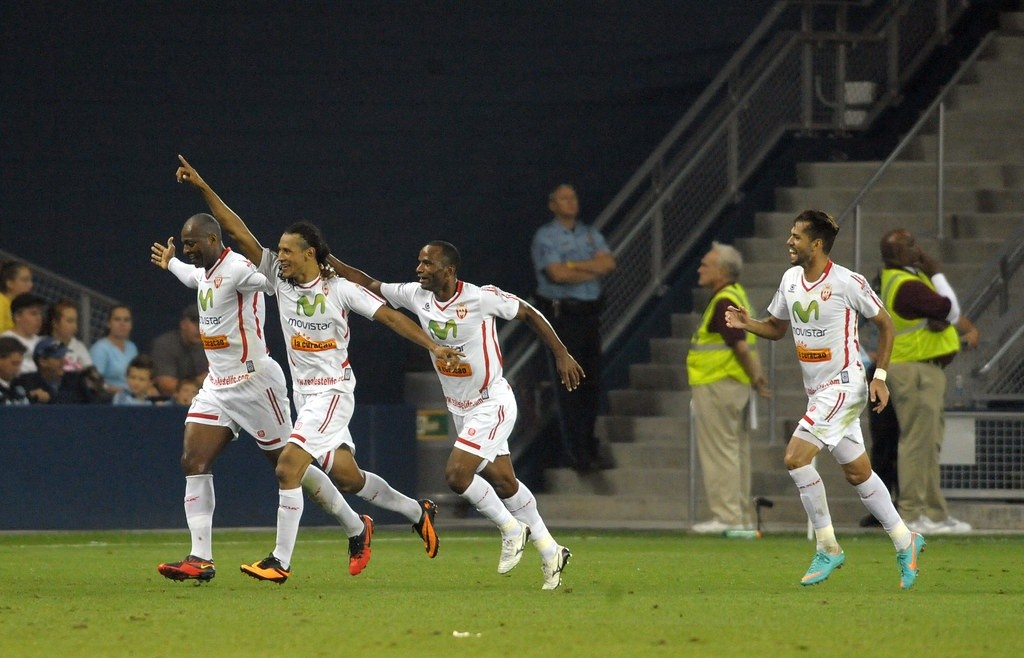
[547,450,613,469]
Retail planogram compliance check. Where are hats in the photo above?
[32,337,74,360]
[182,302,200,319]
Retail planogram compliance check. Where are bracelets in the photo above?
[873,367,888,382]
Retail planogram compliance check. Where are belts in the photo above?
[535,293,597,310]
[918,356,947,368]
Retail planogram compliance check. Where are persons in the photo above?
[0,291,49,374]
[46,296,123,392]
[857,253,980,528]
[531,182,619,476]
[0,255,34,333]
[0,336,51,406]
[88,305,139,394]
[110,351,172,407]
[152,301,209,394]
[9,335,113,406]
[686,241,775,535]
[175,154,466,585]
[156,377,201,408]
[724,208,929,589]
[151,212,373,583]
[325,239,586,591]
[877,228,973,534]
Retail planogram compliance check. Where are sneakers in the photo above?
[413,498,441,558]
[896,529,926,594]
[541,545,574,591]
[799,544,844,585]
[903,516,975,538]
[494,521,532,575]
[238,552,292,585]
[155,555,216,583]
[345,514,375,576]
[692,519,757,534]
